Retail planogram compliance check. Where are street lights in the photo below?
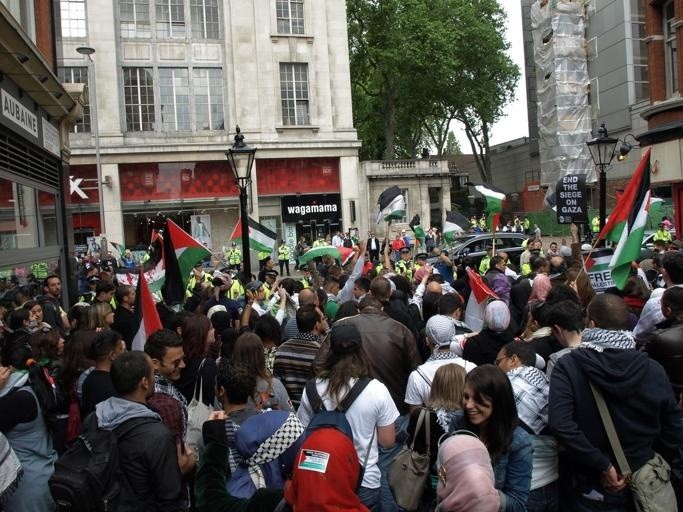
[583,120,618,250]
[222,123,259,308]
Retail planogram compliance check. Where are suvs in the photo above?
[408,231,528,274]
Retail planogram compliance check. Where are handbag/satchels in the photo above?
[628,452,678,512]
[184,356,214,457]
[387,407,431,511]
[65,403,80,441]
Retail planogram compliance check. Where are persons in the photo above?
[0,185,682,511]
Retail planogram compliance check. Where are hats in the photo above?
[415,253,428,260]
[265,270,278,279]
[329,324,361,354]
[483,300,510,332]
[398,247,410,252]
[528,248,540,254]
[245,281,263,292]
[87,275,100,285]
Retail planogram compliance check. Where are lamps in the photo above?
[617,133,639,157]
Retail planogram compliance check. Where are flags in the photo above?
[598,148,652,290]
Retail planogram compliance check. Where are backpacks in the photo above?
[286,375,376,491]
[47,417,159,512]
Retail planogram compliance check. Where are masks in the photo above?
[28,320,38,328]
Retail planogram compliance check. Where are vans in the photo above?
[638,227,675,252]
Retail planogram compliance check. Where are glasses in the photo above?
[486,298,507,305]
[437,430,479,447]
[321,315,327,321]
[493,356,510,367]
[303,287,315,297]
